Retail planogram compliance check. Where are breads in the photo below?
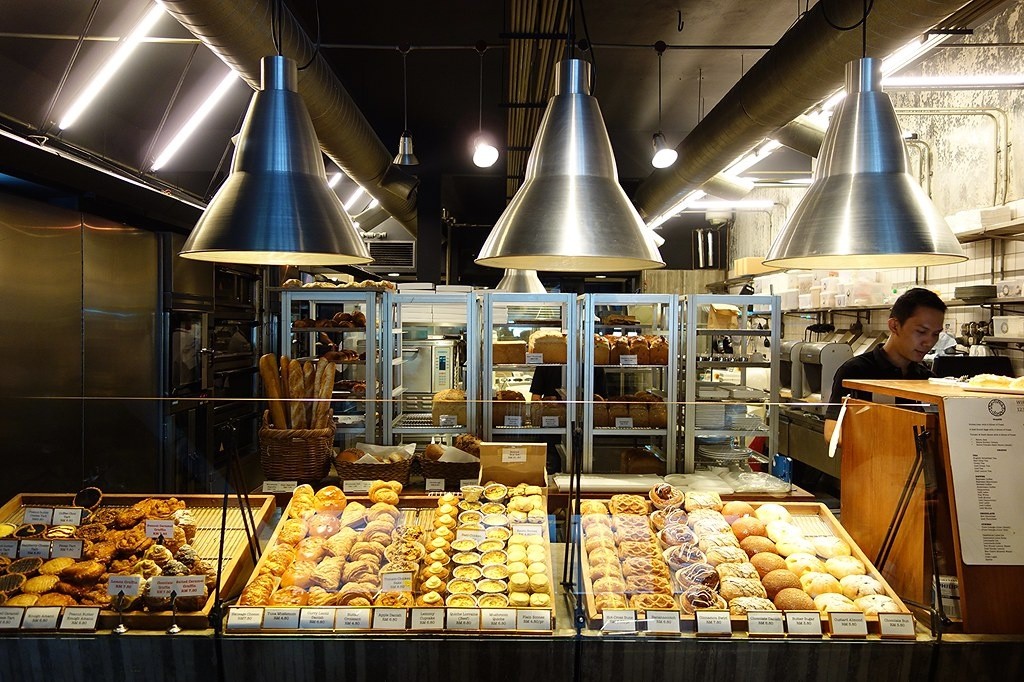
[282,278,397,395]
[240,478,403,606]
[431,314,669,428]
[579,494,679,614]
[685,490,902,618]
[335,433,482,464]
[259,354,336,429]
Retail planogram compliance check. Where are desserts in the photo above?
[0,486,217,611]
[375,483,552,611]
[648,482,729,615]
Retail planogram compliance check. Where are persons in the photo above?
[529,365,607,475]
[823,288,947,448]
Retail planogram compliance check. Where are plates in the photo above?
[696,403,762,431]
[695,434,753,461]
[954,285,997,299]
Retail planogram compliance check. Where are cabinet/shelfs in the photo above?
[281,291,781,474]
[208,272,262,471]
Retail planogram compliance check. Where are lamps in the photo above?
[176,1,377,266]
[472,0,666,272]
[760,0,969,271]
[392,55,420,165]
[471,56,499,168]
[650,54,678,169]
